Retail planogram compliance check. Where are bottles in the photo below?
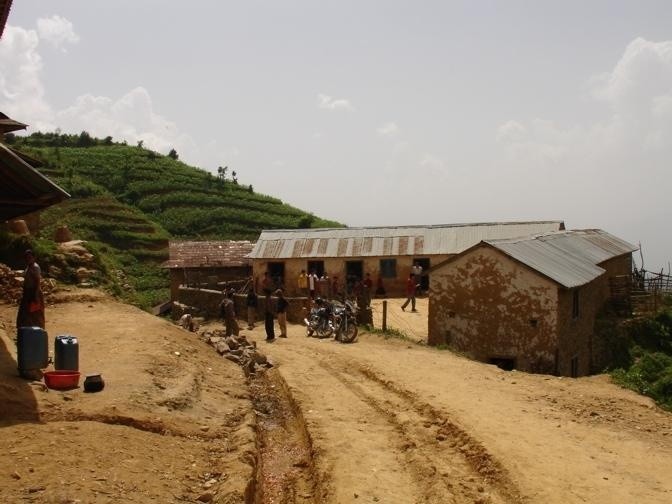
[85,373,106,392]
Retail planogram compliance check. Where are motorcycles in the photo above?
[304,292,360,344]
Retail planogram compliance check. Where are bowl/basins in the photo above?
[45,370,81,389]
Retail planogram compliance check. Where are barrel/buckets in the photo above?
[18,327,47,369]
[55,335,78,370]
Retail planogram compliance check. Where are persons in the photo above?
[412,262,423,295]
[401,274,417,312]
[220,291,239,337]
[275,289,290,338]
[262,272,273,289]
[263,288,275,339]
[298,269,386,307]
[246,287,258,329]
[17,249,46,328]
